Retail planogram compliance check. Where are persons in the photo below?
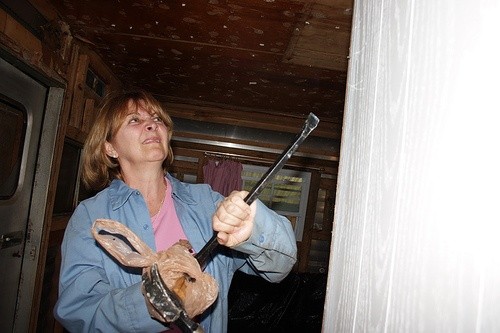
[52,90,298,332]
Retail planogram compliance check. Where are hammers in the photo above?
[140,112,319,333]
